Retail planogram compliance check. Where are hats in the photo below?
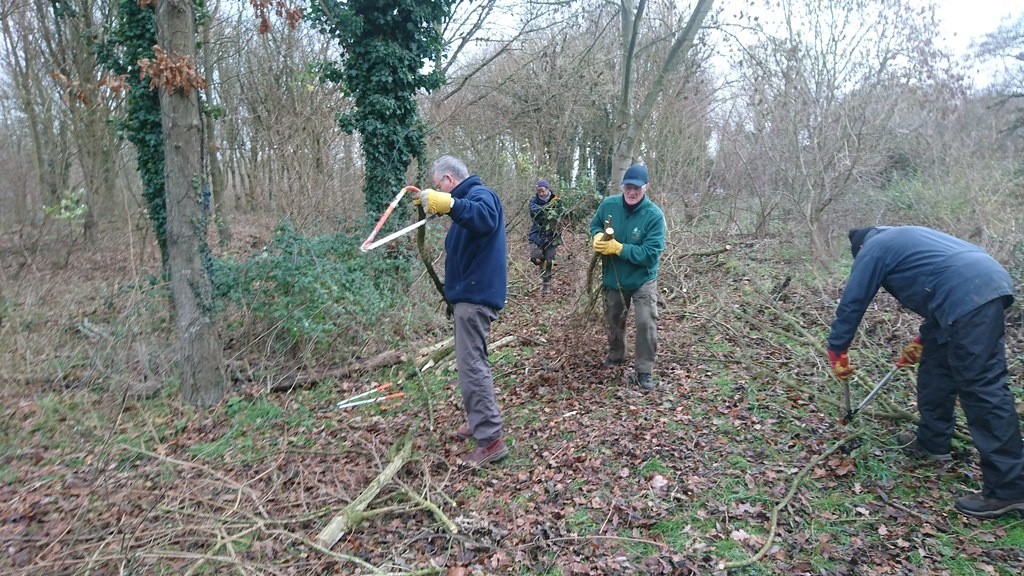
[536,180,550,189]
[848,226,874,260]
[620,164,649,187]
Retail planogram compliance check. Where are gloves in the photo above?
[900,341,924,364]
[592,232,623,257]
[411,187,451,219]
[826,347,857,385]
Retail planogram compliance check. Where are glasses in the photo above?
[621,183,648,193]
[432,173,453,192]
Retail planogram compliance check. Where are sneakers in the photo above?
[540,284,551,294]
[603,358,625,367]
[460,437,510,469]
[897,432,954,461]
[637,372,655,392]
[448,421,474,441]
[954,491,1024,519]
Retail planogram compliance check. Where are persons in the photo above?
[590,165,666,390]
[526,180,563,295]
[413,154,510,467]
[826,226,1024,517]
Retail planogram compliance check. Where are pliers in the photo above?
[841,351,906,426]
[316,382,405,413]
